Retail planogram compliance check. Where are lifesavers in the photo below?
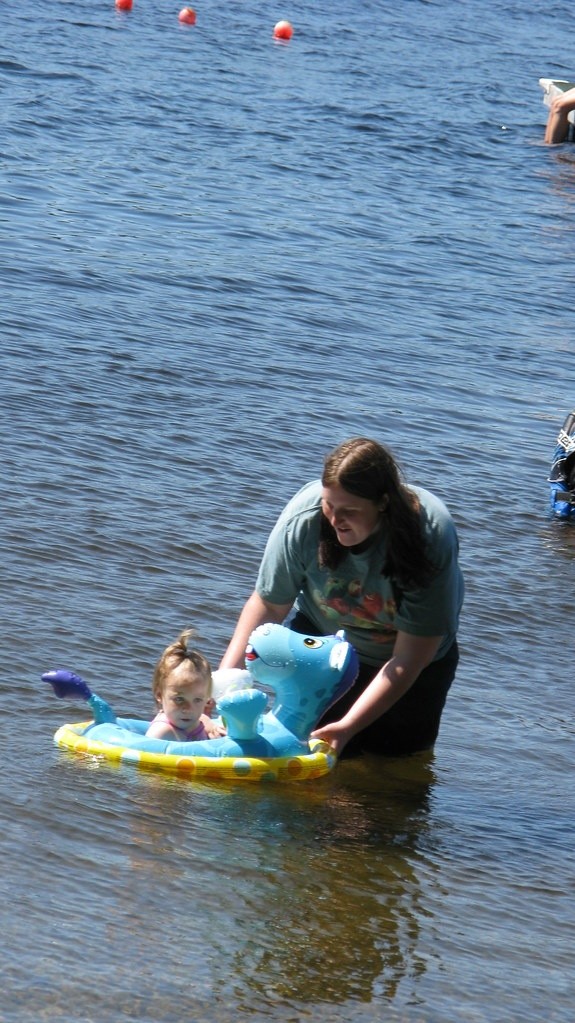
[36,622,362,782]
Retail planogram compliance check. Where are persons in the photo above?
[146,630,226,743]
[544,87,575,145]
[218,438,465,757]
